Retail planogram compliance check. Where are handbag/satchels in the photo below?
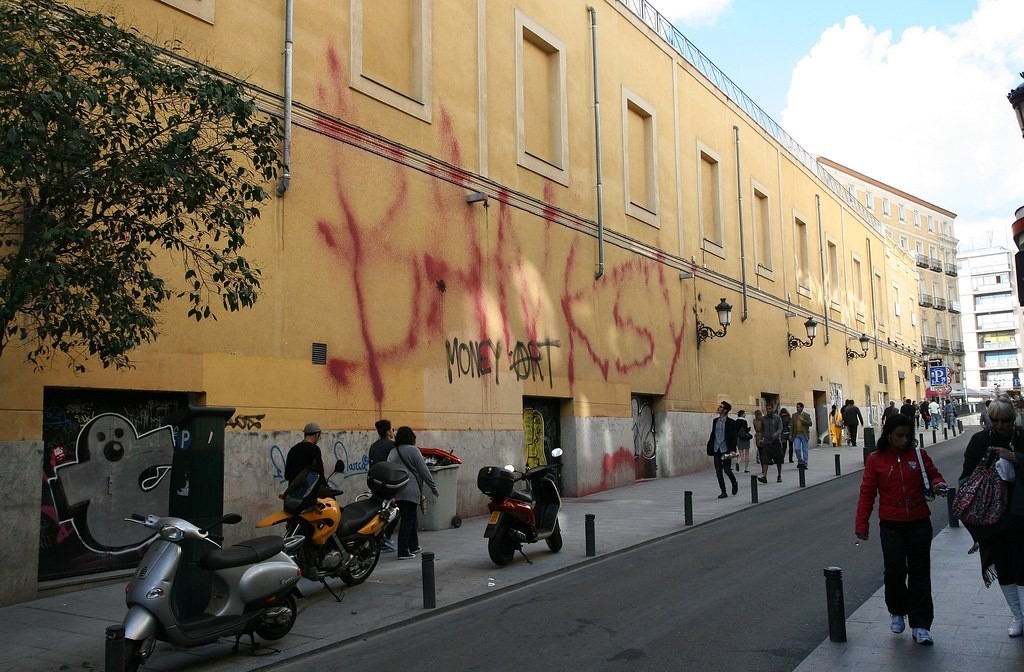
[283,461,321,515]
[952,446,1010,526]
[951,403,959,417]
[421,495,426,515]
[845,428,851,440]
[915,447,936,501]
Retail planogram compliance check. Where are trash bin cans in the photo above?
[416,448,463,531]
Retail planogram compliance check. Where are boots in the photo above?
[1000,583,1024,636]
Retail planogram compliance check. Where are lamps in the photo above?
[696,296,735,350]
[785,316,818,354]
[846,333,870,364]
[910,350,930,373]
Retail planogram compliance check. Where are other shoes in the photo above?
[732,482,738,495]
[736,462,740,471]
[745,470,750,473]
[385,539,396,550]
[797,463,809,469]
[718,494,727,498]
[777,476,782,482]
[758,477,767,483]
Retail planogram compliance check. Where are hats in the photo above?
[302,423,321,433]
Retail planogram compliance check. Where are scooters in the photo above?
[122,513,306,672]
[477,448,563,566]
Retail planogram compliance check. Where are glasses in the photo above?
[719,405,724,408]
[319,436,322,440]
[989,415,1012,423]
[392,428,394,432]
[894,432,912,437]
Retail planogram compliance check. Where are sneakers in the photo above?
[889,613,906,634]
[398,554,416,559]
[912,628,933,645]
[411,545,422,553]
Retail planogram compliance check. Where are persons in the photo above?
[387,426,439,560]
[778,408,796,463]
[980,393,1024,430]
[706,401,738,498]
[369,419,396,552]
[735,409,753,473]
[753,409,767,462]
[829,399,864,447]
[758,403,783,483]
[789,403,812,470]
[958,396,1024,636]
[285,423,329,492]
[882,396,957,430]
[856,414,947,644]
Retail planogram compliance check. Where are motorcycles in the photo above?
[255,459,410,602]
